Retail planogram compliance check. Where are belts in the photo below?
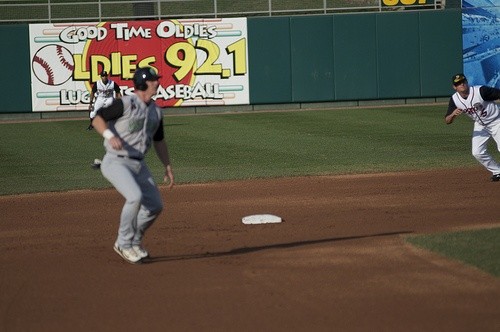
[117,155,143,161]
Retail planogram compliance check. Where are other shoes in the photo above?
[86,125,93,130]
[490,174,500,181]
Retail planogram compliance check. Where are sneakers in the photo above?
[113,241,149,263]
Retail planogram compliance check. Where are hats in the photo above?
[101,71,108,76]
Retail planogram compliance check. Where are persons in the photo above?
[87,71,124,130]
[446,73,500,181]
[92,67,173,264]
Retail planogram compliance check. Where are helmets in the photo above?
[452,73,466,84]
[133,67,162,86]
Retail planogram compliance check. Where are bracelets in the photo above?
[103,130,114,139]
[90,101,92,105]
